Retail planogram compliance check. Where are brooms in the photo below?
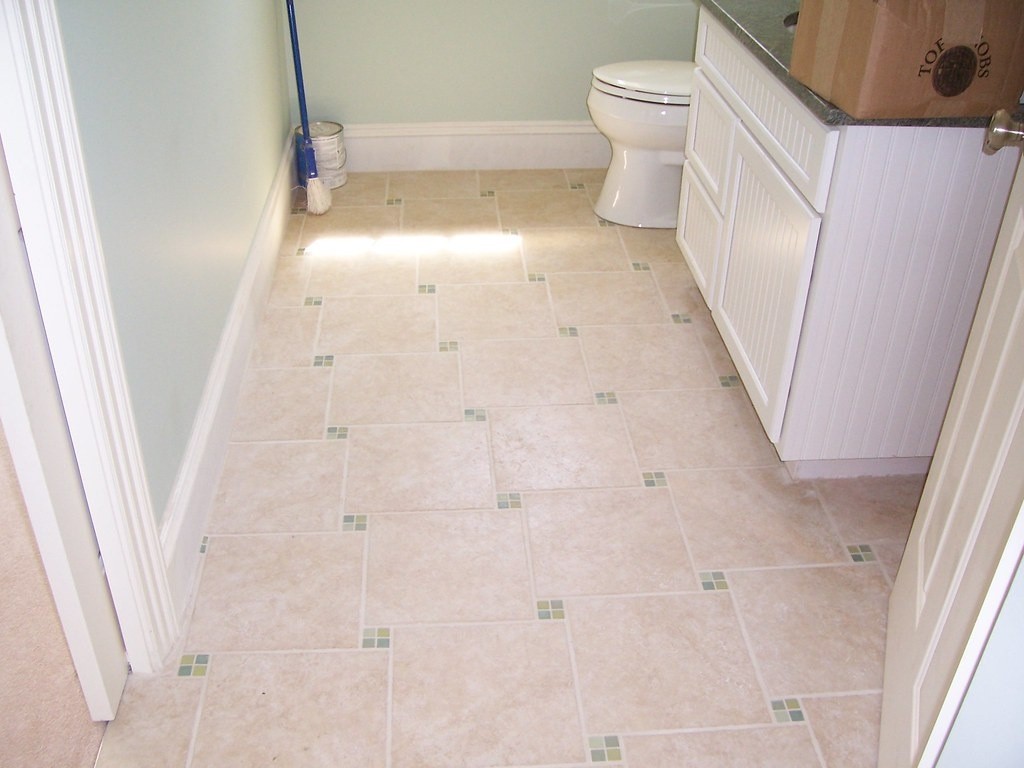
[287,0,334,216]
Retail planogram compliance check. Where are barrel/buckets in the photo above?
[295,121,348,189]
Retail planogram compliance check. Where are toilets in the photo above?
[586,60,700,228]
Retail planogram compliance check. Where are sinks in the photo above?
[781,10,799,36]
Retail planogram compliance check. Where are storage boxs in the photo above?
[787,0,1024,121]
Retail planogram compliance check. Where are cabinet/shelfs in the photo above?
[675,0,1024,476]
